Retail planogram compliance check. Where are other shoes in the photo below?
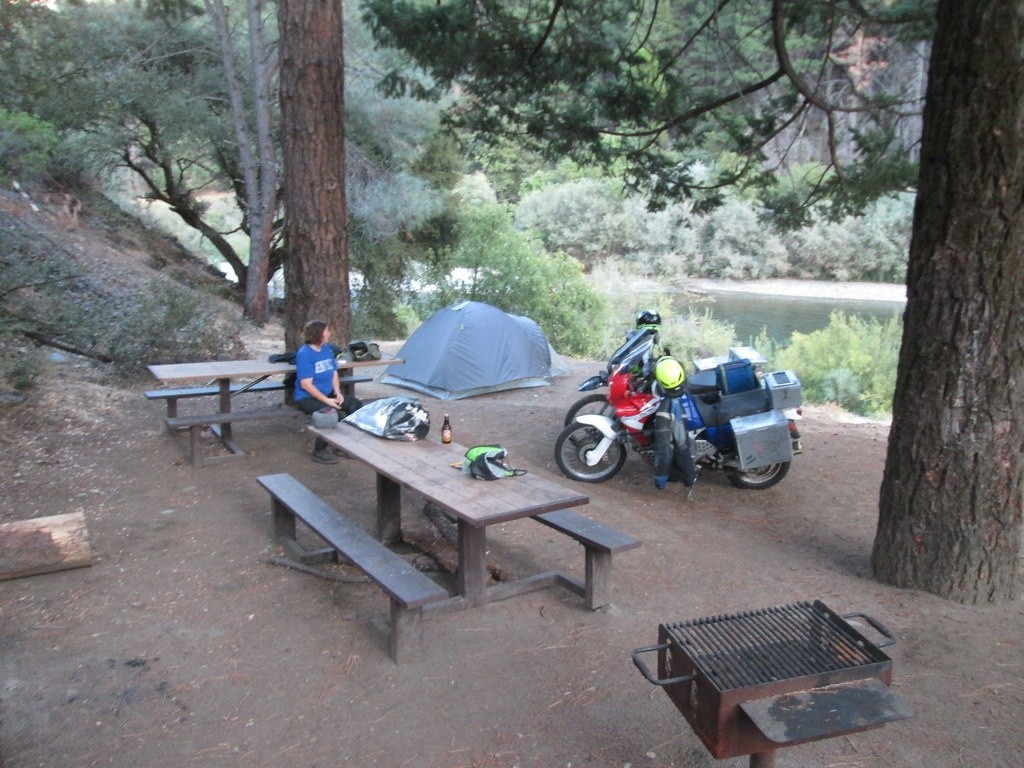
[311,446,357,464]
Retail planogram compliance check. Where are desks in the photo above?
[306,422,590,618]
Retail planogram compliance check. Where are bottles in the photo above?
[442,414,451,444]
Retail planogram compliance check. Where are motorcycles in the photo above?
[563,326,769,447]
[554,335,804,498]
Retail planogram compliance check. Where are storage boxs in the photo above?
[728,347,767,372]
[764,369,802,409]
[692,355,731,375]
[729,408,793,470]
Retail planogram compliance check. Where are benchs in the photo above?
[144,375,372,433]
[146,350,406,463]
[529,509,642,609]
[255,473,449,665]
[166,395,419,469]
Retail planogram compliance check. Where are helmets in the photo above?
[653,356,688,398]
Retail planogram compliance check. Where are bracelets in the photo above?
[334,390,341,394]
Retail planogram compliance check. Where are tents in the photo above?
[377,301,569,401]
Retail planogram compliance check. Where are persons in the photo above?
[293,321,362,464]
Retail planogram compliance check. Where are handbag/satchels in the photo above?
[349,340,381,361]
[340,395,431,441]
[312,407,339,430]
